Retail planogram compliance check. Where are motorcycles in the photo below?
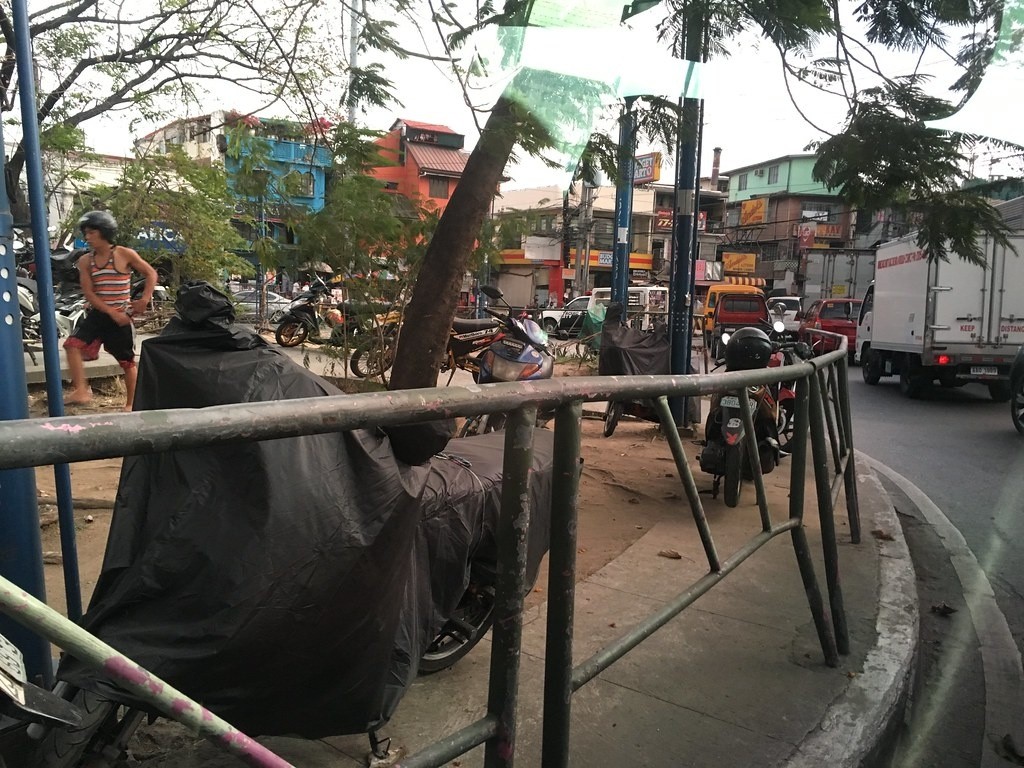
[275,258,561,438]
[12,281,585,764]
[691,302,819,508]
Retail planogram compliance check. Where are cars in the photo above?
[768,296,806,342]
[232,290,294,326]
[536,291,593,340]
[796,298,866,360]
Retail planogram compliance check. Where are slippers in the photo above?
[62,393,91,406]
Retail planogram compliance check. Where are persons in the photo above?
[292,279,309,298]
[529,288,569,309]
[61,210,157,414]
[699,212,705,228]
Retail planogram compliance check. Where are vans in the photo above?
[704,294,773,360]
[700,284,767,351]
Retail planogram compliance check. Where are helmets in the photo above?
[722,326,773,373]
[78,210,119,240]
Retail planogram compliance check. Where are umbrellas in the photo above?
[296,261,395,284]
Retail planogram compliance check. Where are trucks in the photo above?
[800,247,877,319]
[845,225,1023,400]
